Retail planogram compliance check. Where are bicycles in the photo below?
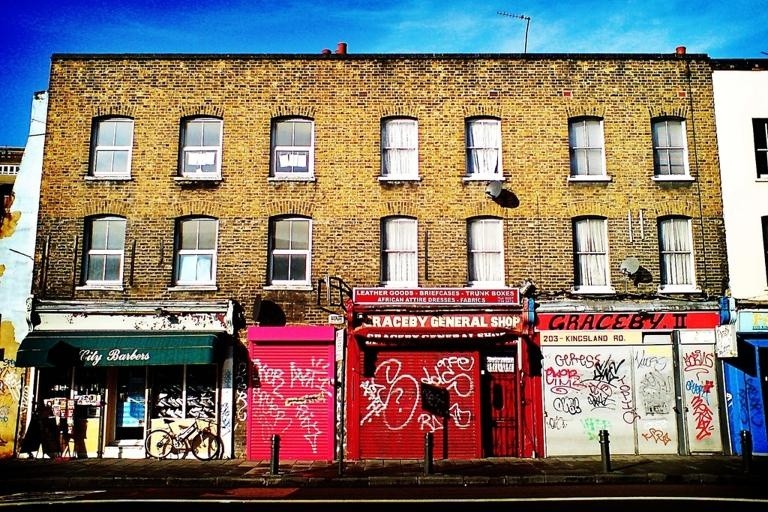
[144,410,221,461]
